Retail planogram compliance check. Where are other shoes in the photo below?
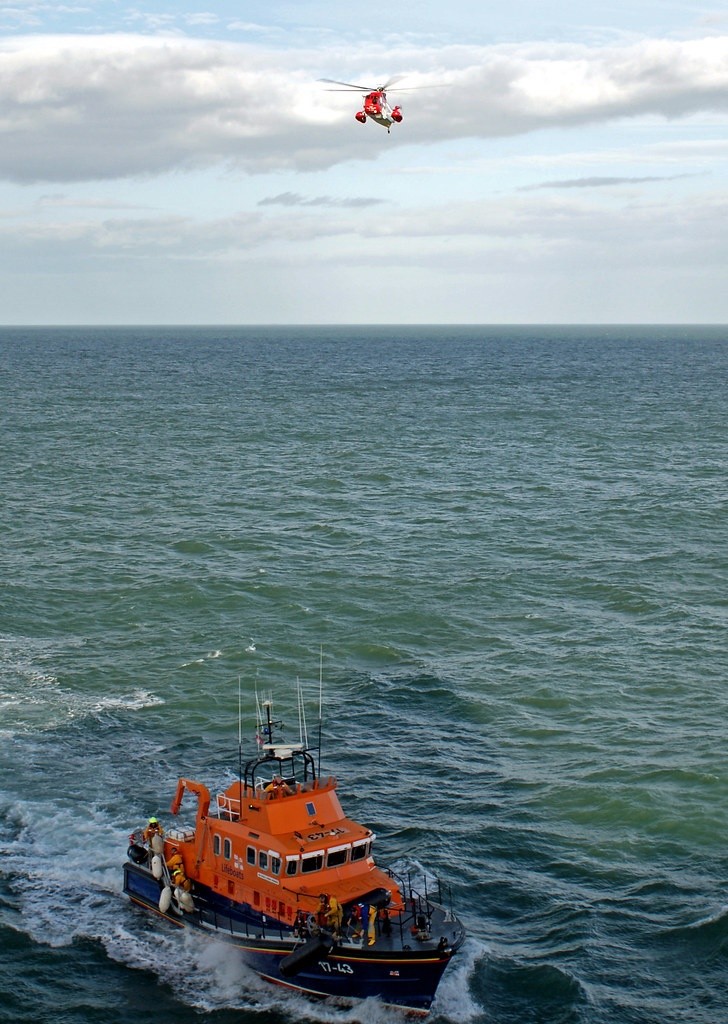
[368,940,375,945]
[352,934,358,937]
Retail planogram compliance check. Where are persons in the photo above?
[171,867,190,893]
[145,817,164,846]
[165,846,184,872]
[263,773,294,800]
[314,893,377,946]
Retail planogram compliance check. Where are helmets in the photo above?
[149,817,157,823]
[173,869,181,876]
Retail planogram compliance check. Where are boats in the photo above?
[120,644,470,1016]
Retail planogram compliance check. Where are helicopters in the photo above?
[312,73,452,135]
